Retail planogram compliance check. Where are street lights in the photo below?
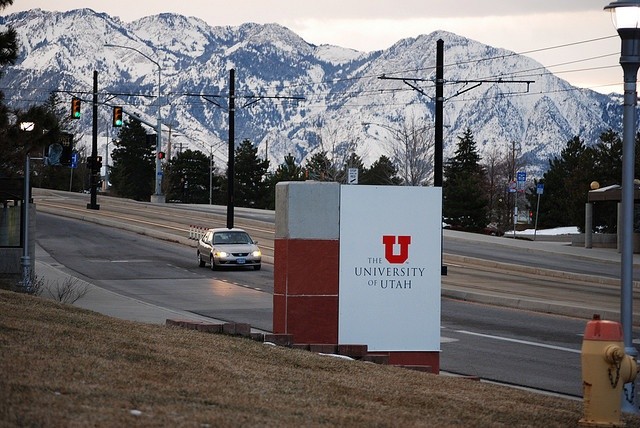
[603,0,639,414]
[102,42,168,202]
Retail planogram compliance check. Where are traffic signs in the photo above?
[348,168,358,184]
[508,189,516,192]
[537,184,544,194]
[517,182,525,193]
[517,171,526,182]
[42,129,73,166]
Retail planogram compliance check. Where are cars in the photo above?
[197,228,261,270]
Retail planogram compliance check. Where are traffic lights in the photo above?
[72,98,81,119]
[113,106,122,126]
[158,151,166,159]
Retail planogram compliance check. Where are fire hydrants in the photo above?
[579,314,638,427]
